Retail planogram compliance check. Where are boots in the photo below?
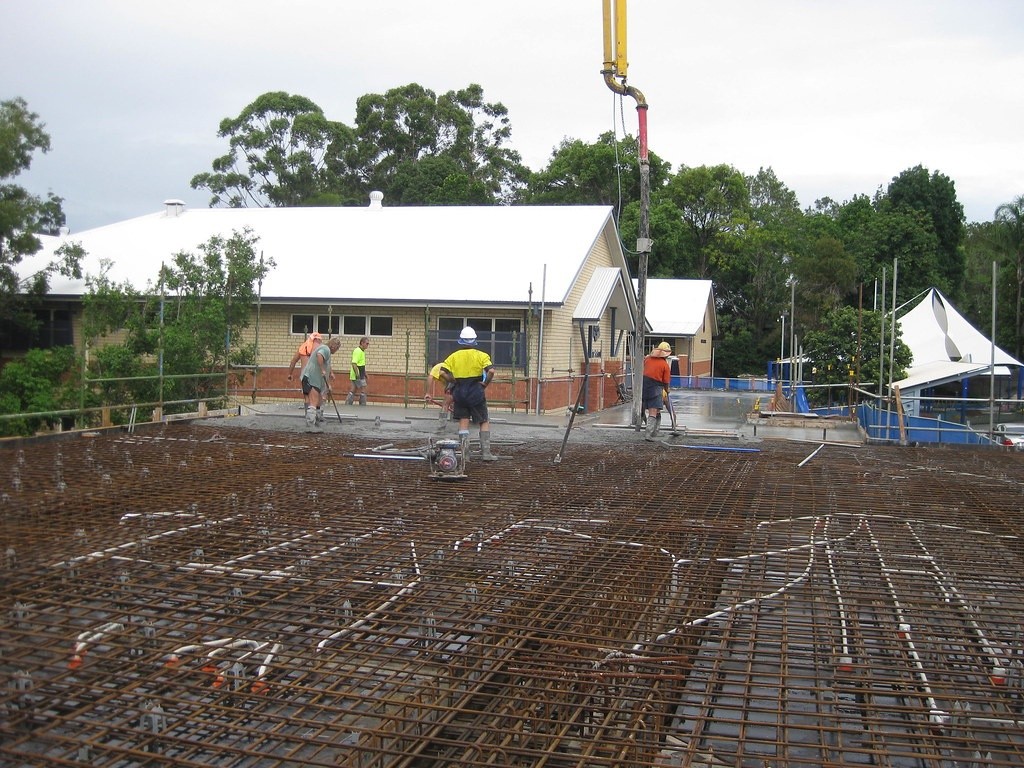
[359,393,366,405]
[458,433,471,465]
[439,413,448,421]
[345,393,355,405]
[652,413,664,436]
[479,431,498,460]
[645,417,654,442]
[304,403,310,412]
[305,410,324,433]
[316,409,326,421]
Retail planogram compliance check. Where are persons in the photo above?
[300,337,341,433]
[345,337,369,405]
[642,342,673,441]
[425,326,498,460]
[287,332,320,413]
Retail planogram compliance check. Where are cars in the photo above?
[986,423,1024,449]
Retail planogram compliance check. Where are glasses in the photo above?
[363,341,369,345]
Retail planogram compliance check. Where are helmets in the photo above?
[658,342,671,351]
[459,326,477,338]
